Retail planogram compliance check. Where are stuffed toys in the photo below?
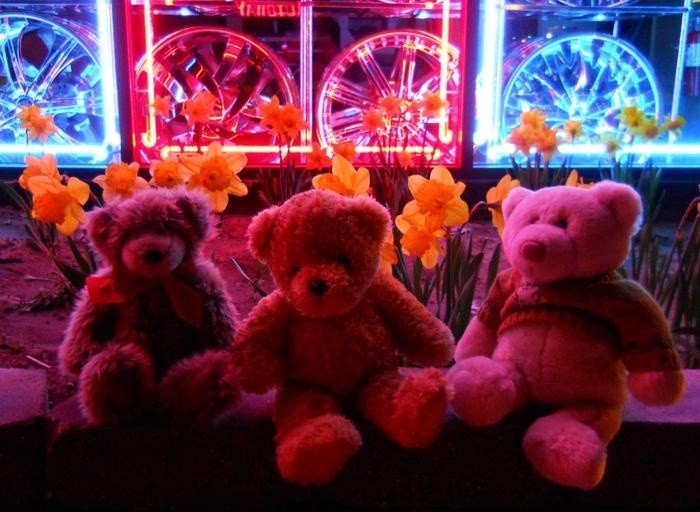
[444,180,685,488]
[231,188,456,483]
[58,187,242,426]
